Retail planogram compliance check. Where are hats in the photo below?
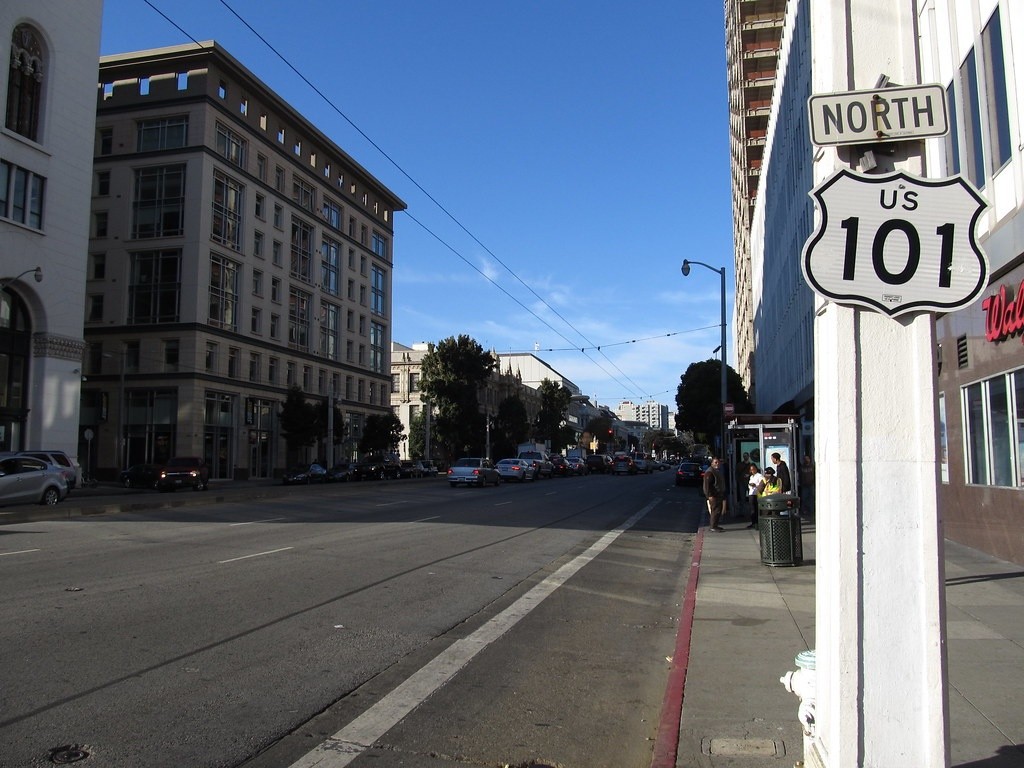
[764,467,775,474]
[773,453,780,459]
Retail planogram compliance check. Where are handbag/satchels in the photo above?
[706,499,727,516]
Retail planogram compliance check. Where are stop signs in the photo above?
[724,404,734,414]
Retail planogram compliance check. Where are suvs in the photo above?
[354,453,402,480]
[1,450,75,489]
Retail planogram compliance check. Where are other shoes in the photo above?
[710,527,726,532]
[747,522,755,528]
[754,523,759,529]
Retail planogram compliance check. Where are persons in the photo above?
[757,467,782,498]
[771,453,792,495]
[703,457,726,533]
[799,455,815,521]
[742,453,751,498]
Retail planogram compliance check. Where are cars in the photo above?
[283,464,327,485]
[447,457,500,488]
[400,460,424,478]
[326,463,362,483]
[159,457,209,491]
[120,464,159,490]
[664,443,712,487]
[0,456,68,507]
[494,459,537,482]
[517,443,681,478]
[421,460,438,477]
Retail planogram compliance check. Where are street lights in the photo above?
[486,411,494,459]
[681,259,726,404]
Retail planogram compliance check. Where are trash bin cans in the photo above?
[757,494,804,568]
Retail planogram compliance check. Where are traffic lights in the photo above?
[609,429,612,437]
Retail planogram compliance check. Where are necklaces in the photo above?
[747,463,765,531]
